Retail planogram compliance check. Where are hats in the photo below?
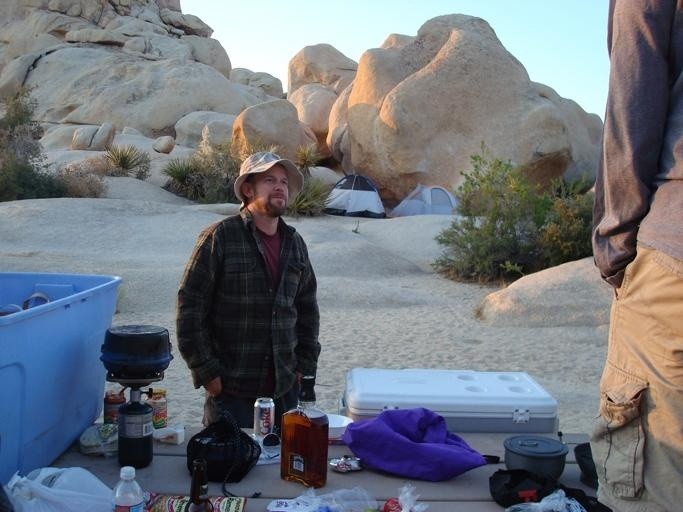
[234,152,304,213]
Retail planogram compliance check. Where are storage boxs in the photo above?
[338,367,560,436]
[0,266,121,481]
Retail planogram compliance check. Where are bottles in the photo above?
[280,375,330,489]
[104,390,126,425]
[144,389,167,428]
[111,466,144,511]
[253,397,275,437]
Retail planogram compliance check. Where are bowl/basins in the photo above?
[329,414,354,439]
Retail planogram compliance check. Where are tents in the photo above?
[387,184,462,218]
[323,175,386,219]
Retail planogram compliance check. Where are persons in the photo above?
[593,0,683,512]
[177,151,320,428]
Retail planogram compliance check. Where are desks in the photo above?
[14,425,601,511]
